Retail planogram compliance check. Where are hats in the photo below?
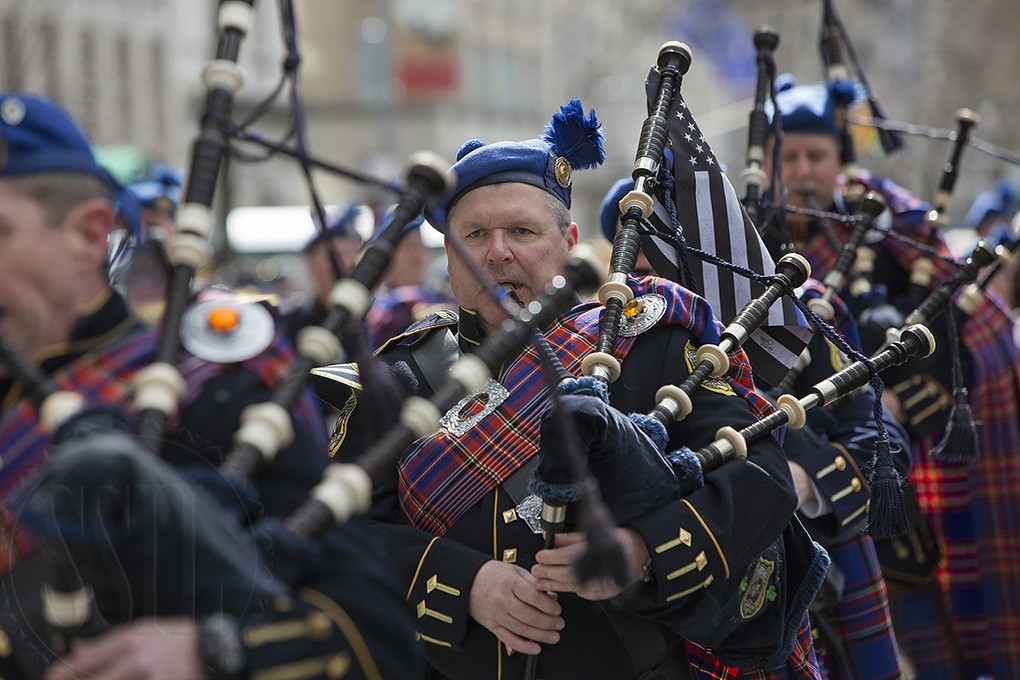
[602,177,636,243]
[426,98,605,233]
[766,74,857,135]
[123,168,183,207]
[369,199,427,245]
[302,204,376,254]
[0,92,107,178]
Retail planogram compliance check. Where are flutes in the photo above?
[496,37,937,594]
[738,24,1019,460]
[2,0,609,677]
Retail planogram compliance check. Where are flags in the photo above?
[649,74,811,387]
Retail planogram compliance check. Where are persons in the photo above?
[0,93,420,680]
[312,102,798,680]
[107,79,1020,680]
